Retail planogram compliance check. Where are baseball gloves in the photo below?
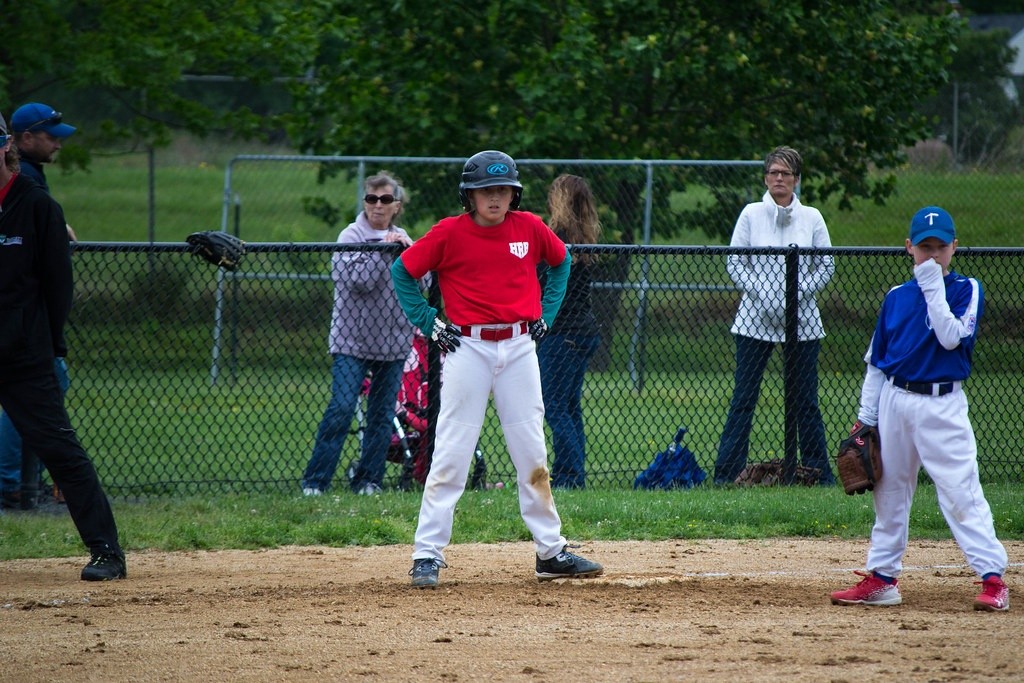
[185,229,247,271]
[830,419,883,496]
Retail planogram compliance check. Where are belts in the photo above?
[461,322,527,340]
[886,375,952,395]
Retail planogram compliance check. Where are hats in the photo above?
[10,101,77,137]
[911,206,955,246]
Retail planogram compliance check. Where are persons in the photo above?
[828,205,1011,614]
[298,172,432,499]
[0,122,126,582]
[388,150,603,588]
[0,102,79,512]
[534,174,601,489]
[712,146,837,488]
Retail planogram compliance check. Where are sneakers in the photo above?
[973,576,1010,611]
[81,546,126,582]
[830,570,902,606]
[408,557,447,588]
[535,545,603,581]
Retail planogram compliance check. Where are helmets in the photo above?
[458,150,522,212]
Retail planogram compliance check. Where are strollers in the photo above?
[344,326,487,491]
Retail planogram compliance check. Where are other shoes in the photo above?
[21,495,38,510]
[360,482,383,496]
[0,490,21,509]
[303,487,321,496]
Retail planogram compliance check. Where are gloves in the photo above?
[430,315,461,353]
[528,317,549,342]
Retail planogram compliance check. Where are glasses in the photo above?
[363,194,397,205]
[27,113,62,130]
[767,168,794,178]
